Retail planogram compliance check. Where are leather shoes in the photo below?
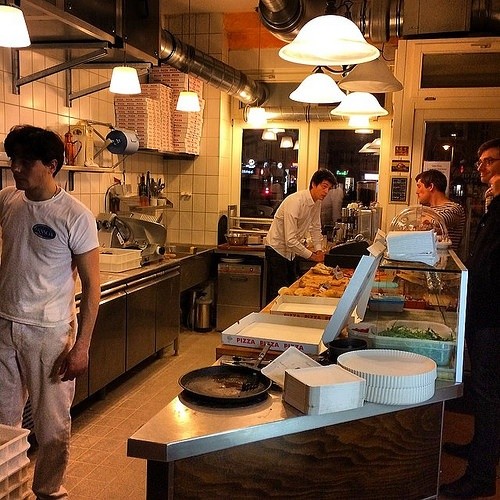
[440,469,496,498]
[444,441,477,460]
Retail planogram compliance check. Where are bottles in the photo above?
[340,207,358,235]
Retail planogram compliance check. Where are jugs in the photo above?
[64,133,82,165]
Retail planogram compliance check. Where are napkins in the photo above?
[386,229,440,266]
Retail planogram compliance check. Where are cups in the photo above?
[150,199,166,207]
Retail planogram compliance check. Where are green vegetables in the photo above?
[376,320,457,364]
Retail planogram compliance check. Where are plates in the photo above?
[178,364,272,400]
[222,257,242,263]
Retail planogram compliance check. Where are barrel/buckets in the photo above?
[182,289,213,330]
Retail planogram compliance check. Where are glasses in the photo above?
[475,158,500,169]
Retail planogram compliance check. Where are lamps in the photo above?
[278,0,404,128]
[0,0,31,48]
[261,130,299,150]
[109,51,141,94]
[358,138,381,152]
[176,0,200,112]
[248,18,267,126]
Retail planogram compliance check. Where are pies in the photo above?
[285,263,354,298]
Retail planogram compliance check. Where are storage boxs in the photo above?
[114,63,206,155]
[99,248,142,273]
[0,423,33,500]
[269,228,385,325]
[221,241,386,356]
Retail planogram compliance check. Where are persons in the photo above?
[465,138,500,433]
[321,182,345,227]
[414,169,466,254]
[264,168,337,306]
[0,125,101,500]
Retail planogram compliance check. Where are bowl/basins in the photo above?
[328,338,367,358]
[225,233,247,245]
[369,296,405,312]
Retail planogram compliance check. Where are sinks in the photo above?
[165,246,217,286]
[165,252,192,292]
